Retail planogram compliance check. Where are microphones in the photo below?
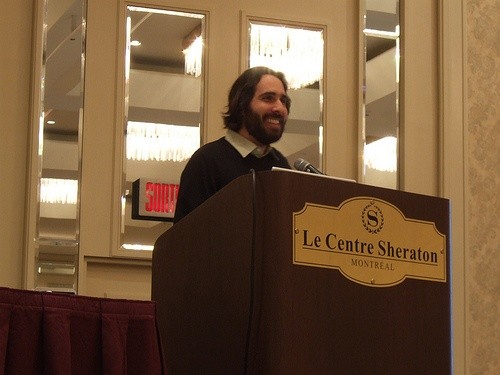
[294,158,323,175]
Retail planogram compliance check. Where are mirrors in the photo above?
[35,0,404,294]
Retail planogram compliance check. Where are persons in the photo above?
[173,66,292,226]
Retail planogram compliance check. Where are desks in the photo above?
[0,286,167,375]
